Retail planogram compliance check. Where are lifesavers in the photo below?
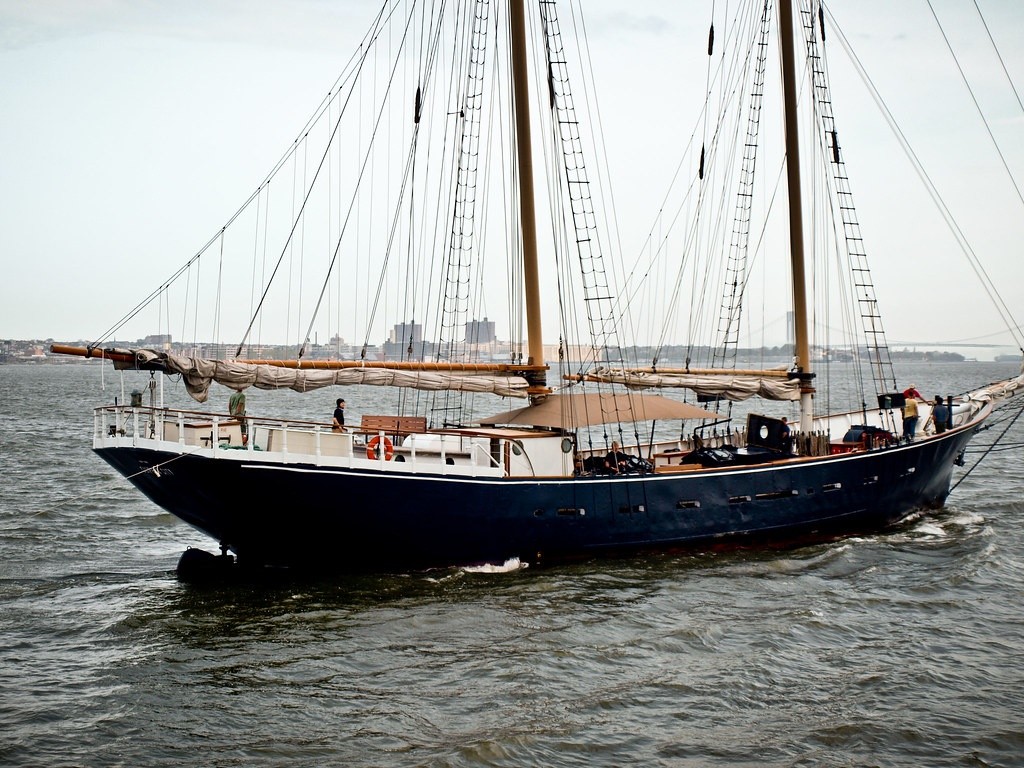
[368,437,394,459]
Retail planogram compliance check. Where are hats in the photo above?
[910,383,915,388]
[336,398,346,408]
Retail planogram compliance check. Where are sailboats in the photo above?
[50,1,1024,574]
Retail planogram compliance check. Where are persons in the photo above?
[331,398,348,431]
[685,433,693,449]
[780,416,791,445]
[899,383,950,443]
[228,387,250,444]
[605,441,626,478]
